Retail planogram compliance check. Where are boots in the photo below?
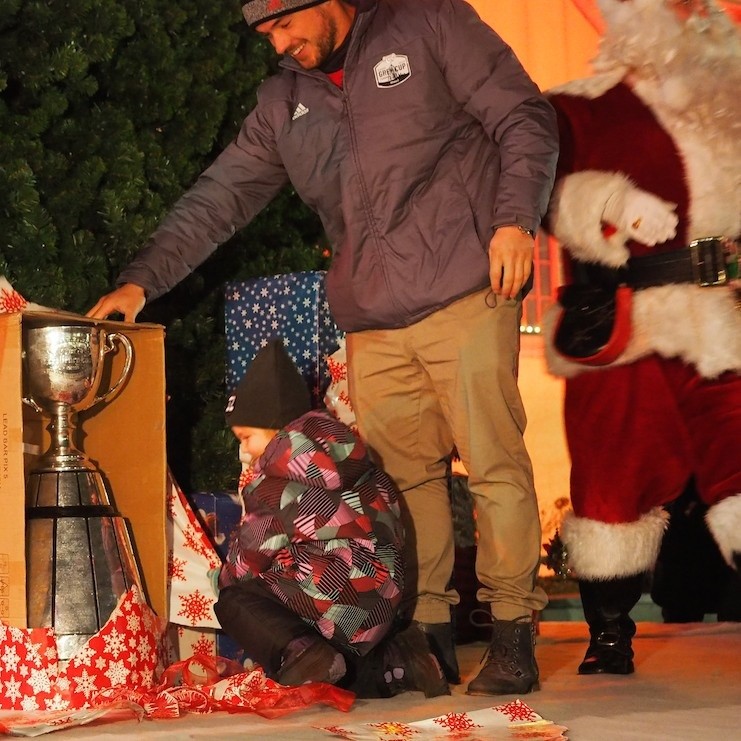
[405,623,462,686]
[576,570,645,675]
[466,620,540,696]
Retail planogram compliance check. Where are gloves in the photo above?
[602,188,680,247]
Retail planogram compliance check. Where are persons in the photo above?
[538,0,740,677]
[82,0,562,698]
[212,332,452,701]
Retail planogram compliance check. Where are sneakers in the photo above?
[386,620,451,698]
[271,636,347,687]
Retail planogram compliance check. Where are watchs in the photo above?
[518,226,533,235]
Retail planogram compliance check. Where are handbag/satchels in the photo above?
[551,281,635,366]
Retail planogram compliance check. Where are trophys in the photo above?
[22,324,134,474]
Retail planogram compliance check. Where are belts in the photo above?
[627,236,732,290]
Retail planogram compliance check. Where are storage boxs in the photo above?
[221,269,345,413]
[0,309,169,635]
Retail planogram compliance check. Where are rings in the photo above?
[632,216,642,230]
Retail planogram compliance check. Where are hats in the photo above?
[224,339,311,430]
[239,0,332,31]
